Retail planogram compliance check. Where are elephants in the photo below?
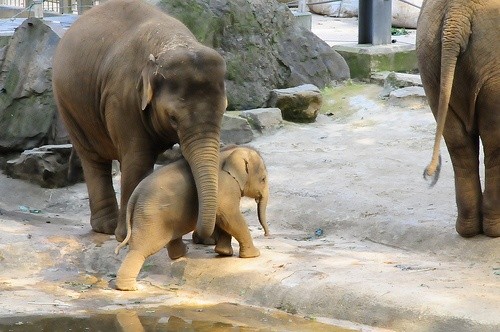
[51,0,227,243]
[112,144,270,292]
[415,0,500,238]
